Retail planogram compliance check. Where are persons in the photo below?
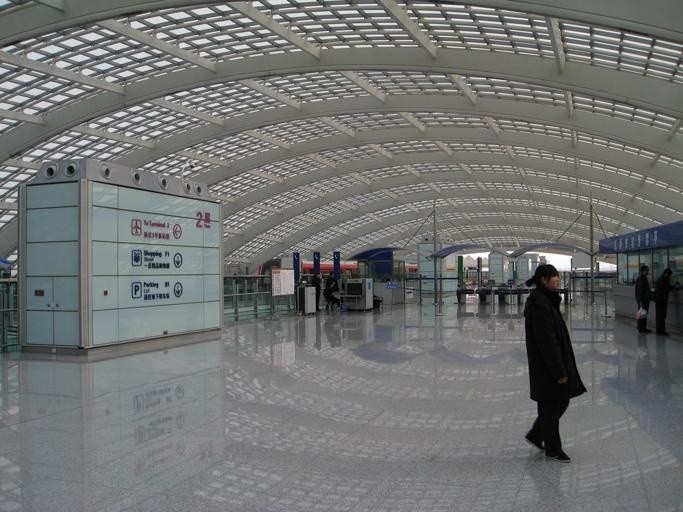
[652,267,677,336]
[635,335,651,396]
[653,337,676,402]
[633,263,653,334]
[322,271,338,308]
[521,262,587,464]
[322,310,340,348]
[314,317,321,352]
[311,272,322,313]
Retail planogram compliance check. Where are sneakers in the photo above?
[524,430,544,452]
[656,330,670,336]
[638,327,652,334]
[545,447,572,464]
